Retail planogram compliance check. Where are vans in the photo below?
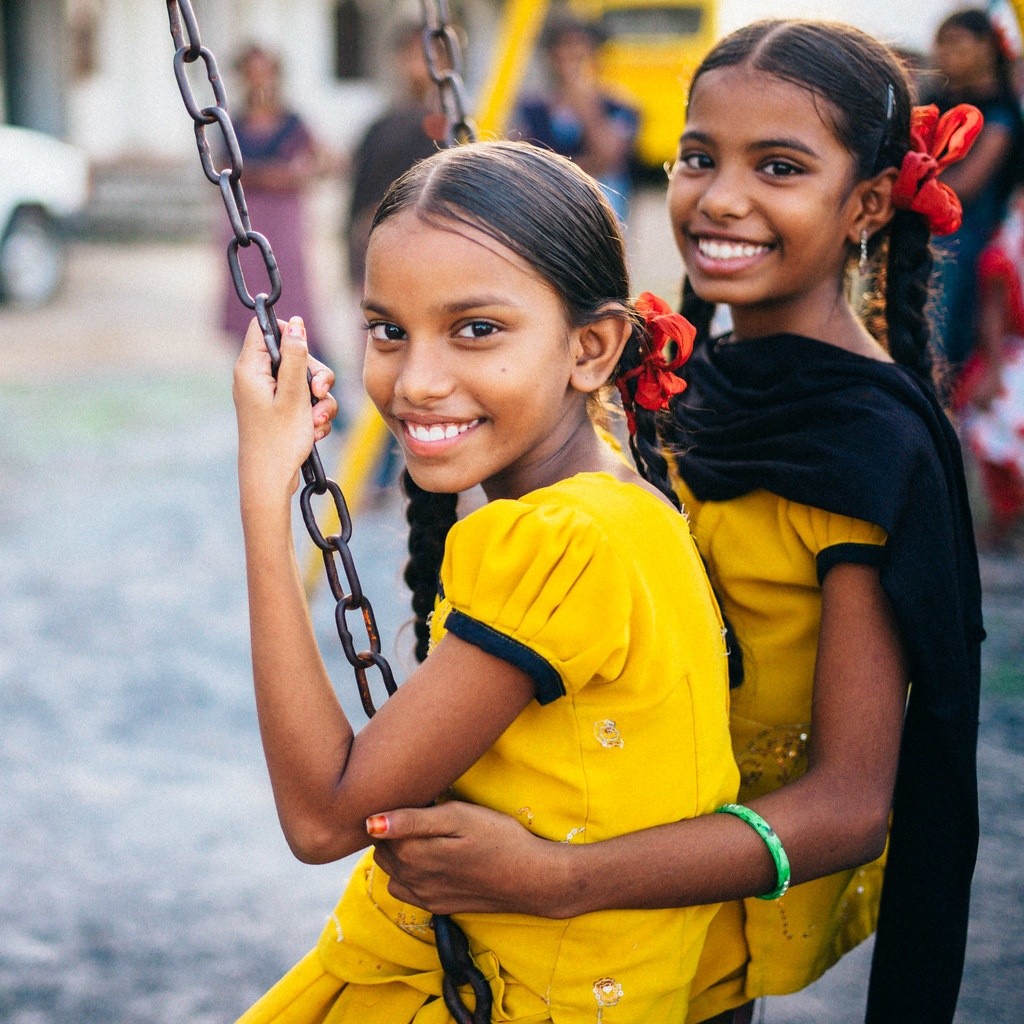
[572,0,773,172]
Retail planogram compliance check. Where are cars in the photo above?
[1,122,93,307]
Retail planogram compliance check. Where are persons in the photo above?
[367,20,989,1023]
[233,142,740,1024]
[909,10,1023,409]
[209,20,638,508]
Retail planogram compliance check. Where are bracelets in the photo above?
[714,803,791,900]
